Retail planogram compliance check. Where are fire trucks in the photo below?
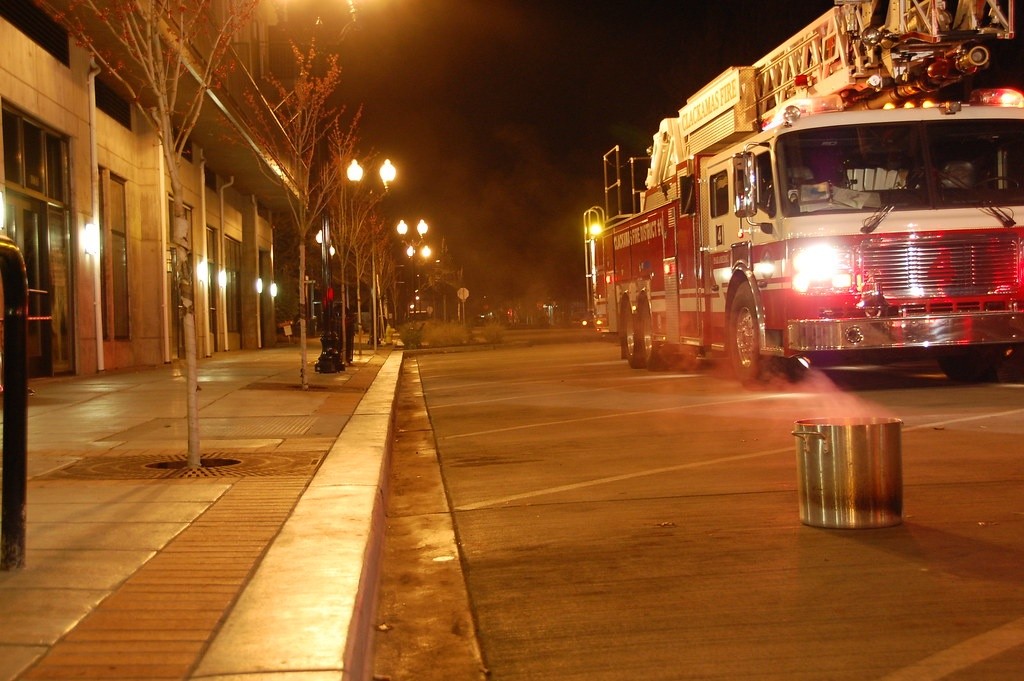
[583,0,1024,392]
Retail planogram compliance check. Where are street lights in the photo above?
[406,245,432,316]
[345,157,398,356]
[394,217,430,323]
[314,230,336,334]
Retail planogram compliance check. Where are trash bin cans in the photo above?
[331,303,354,366]
[0,234,30,571]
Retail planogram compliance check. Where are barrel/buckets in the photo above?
[790,417,904,529]
